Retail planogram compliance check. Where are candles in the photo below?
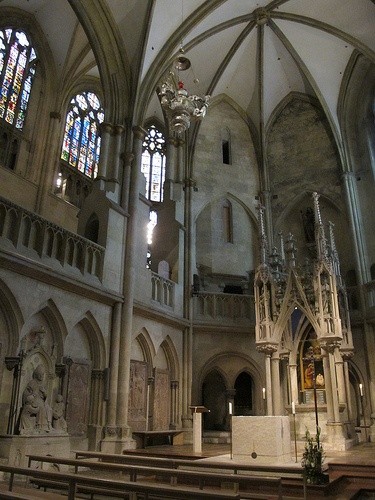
[292,401,296,414]
[229,402,232,414]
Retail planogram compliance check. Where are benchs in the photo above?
[0,448,311,500]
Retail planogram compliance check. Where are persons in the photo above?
[304,363,314,388]
[18,366,67,430]
